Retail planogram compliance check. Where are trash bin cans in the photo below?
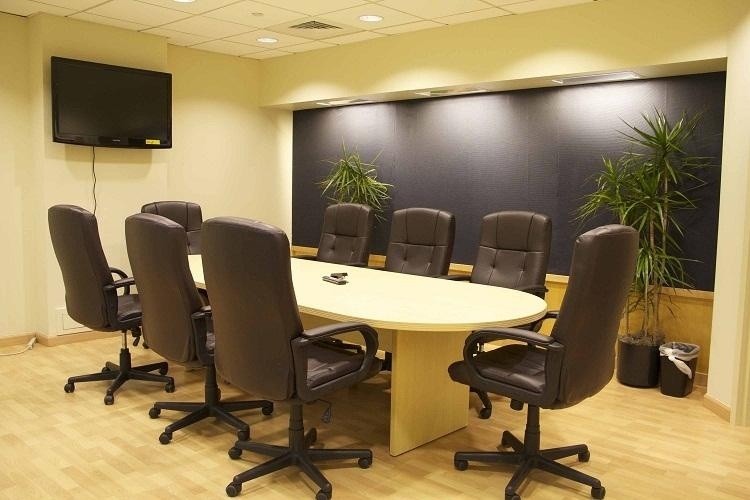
[659,340,701,398]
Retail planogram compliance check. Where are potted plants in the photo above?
[567,104,721,390]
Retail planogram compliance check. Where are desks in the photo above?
[157,252,550,458]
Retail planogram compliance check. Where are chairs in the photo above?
[196,215,383,500]
[142,199,202,255]
[47,203,176,406]
[314,203,376,268]
[447,224,640,500]
[437,209,551,301]
[124,212,274,445]
[382,206,455,279]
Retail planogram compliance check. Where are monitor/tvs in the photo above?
[51,56,173,148]
